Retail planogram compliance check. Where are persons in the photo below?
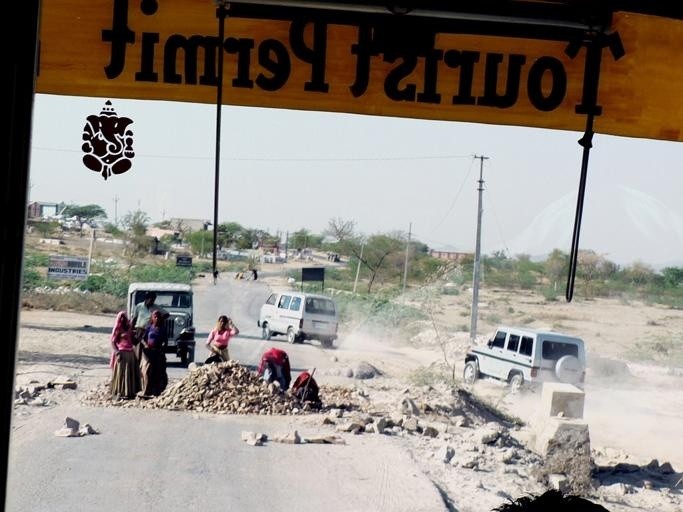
[255,346,290,395]
[139,307,169,397]
[128,290,170,370]
[204,313,239,363]
[212,267,218,285]
[108,311,147,403]
[251,268,257,280]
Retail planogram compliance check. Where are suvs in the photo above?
[462,325,587,399]
[127,281,196,366]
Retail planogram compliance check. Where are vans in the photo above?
[257,291,338,349]
